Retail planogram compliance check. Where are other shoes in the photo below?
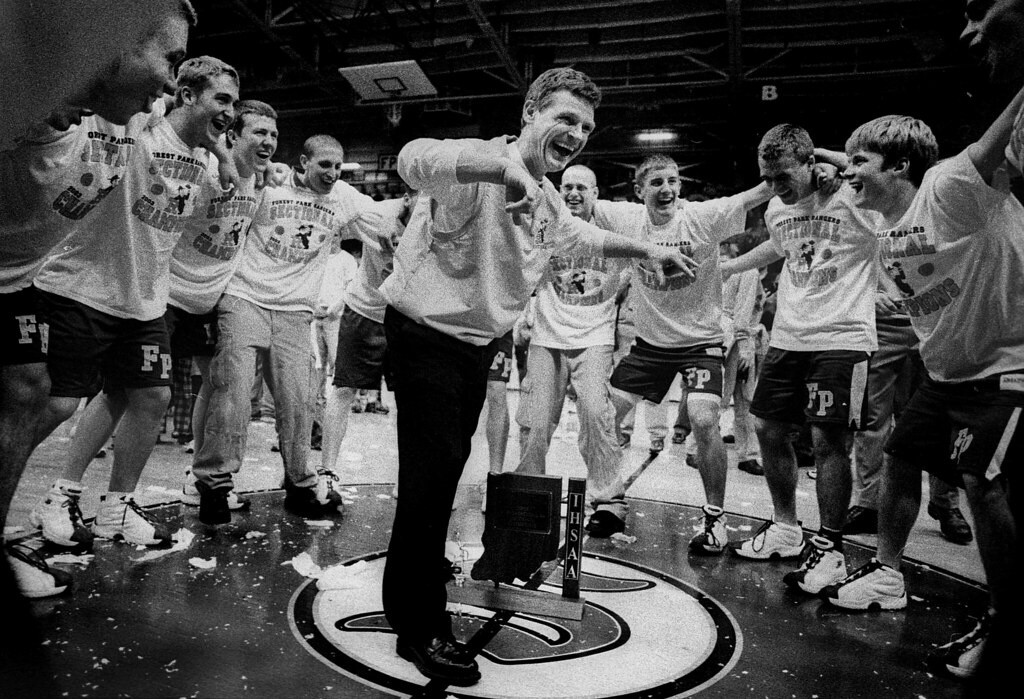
[672,432,685,443]
[195,484,231,527]
[685,454,698,467]
[282,487,343,523]
[365,401,390,414]
[584,512,627,535]
[184,439,196,452]
[738,460,765,475]
[808,468,816,478]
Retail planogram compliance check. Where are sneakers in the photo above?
[0,540,70,598]
[687,504,730,552]
[727,517,805,560]
[315,471,343,506]
[818,559,907,613]
[181,473,252,510]
[91,496,171,546]
[29,486,91,548]
[944,608,1003,675]
[784,536,849,593]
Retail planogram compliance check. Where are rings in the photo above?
[527,196,536,202]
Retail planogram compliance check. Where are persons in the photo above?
[0,0,430,598]
[376,68,697,687]
[481,87,1024,590]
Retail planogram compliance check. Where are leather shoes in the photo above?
[928,503,972,543]
[844,506,878,532]
[397,630,481,687]
